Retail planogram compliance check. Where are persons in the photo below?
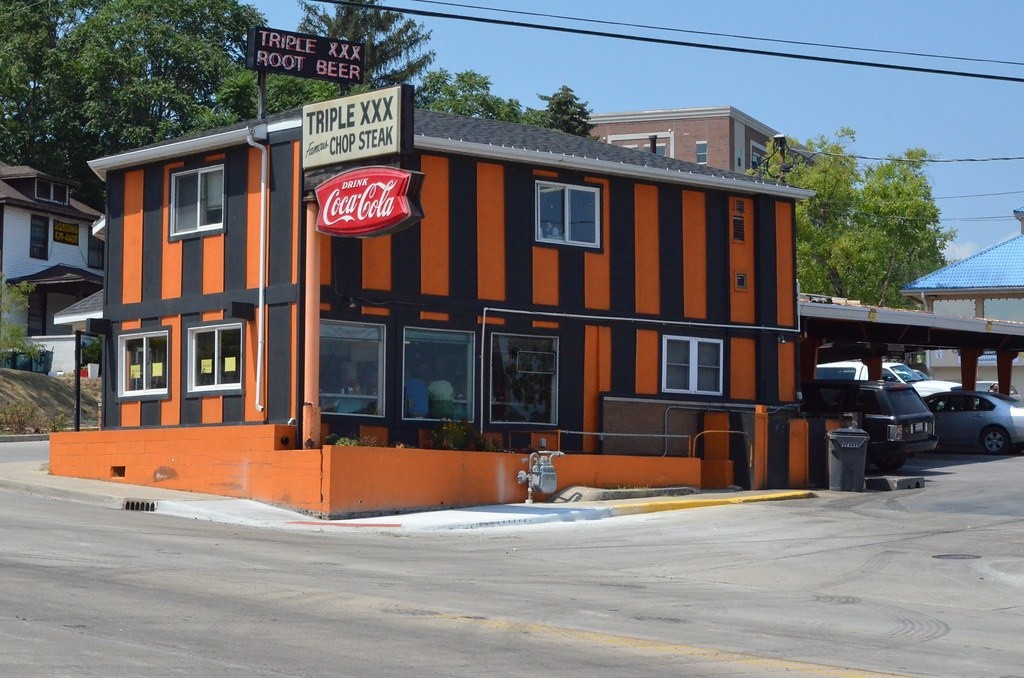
[405,369,465,420]
[346,373,366,395]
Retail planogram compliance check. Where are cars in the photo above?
[975,381,1021,401]
[894,370,931,380]
[922,390,1024,454]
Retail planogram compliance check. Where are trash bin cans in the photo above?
[88,363,99,379]
[0,351,16,368]
[827,428,871,491]
[14,351,32,370]
[31,349,53,375]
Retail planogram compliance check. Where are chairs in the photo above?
[335,397,372,415]
[428,399,456,419]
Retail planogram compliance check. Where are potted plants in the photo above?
[79,333,103,378]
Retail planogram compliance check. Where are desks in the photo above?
[319,394,550,423]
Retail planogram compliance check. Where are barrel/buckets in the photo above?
[87,363,99,378]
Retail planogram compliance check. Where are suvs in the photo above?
[802,379,937,469]
[817,361,962,397]
